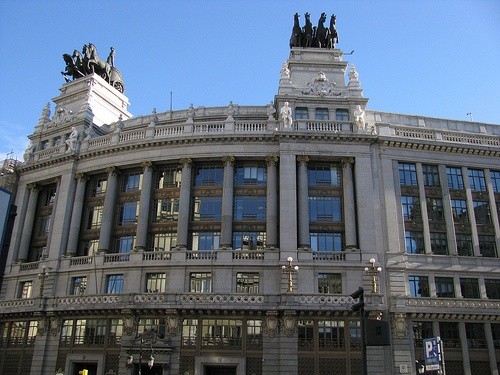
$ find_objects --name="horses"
[289,12,339,50]
[60,43,112,86]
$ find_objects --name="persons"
[107,46,116,68]
[22,140,35,165]
[65,126,79,152]
[280,101,293,129]
[353,105,366,131]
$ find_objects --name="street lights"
[126,338,154,375]
[352,286,368,374]
